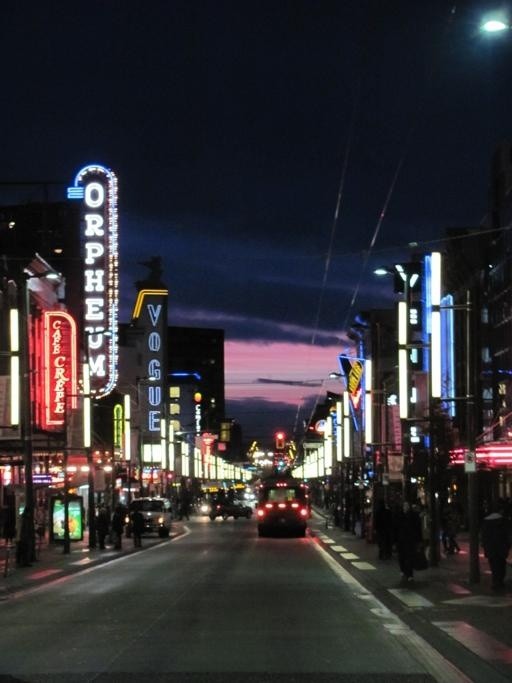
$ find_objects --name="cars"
[125,497,171,538]
[209,497,252,520]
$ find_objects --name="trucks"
[256,482,308,537]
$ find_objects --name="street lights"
[16,269,62,561]
[82,330,113,547]
[371,265,414,502]
[136,375,157,496]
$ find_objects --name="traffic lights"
[275,431,285,449]
[192,392,202,403]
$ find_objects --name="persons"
[179,492,190,520]
[372,499,422,586]
[482,505,509,590]
[442,519,460,555]
[20,512,34,567]
[91,502,146,552]
